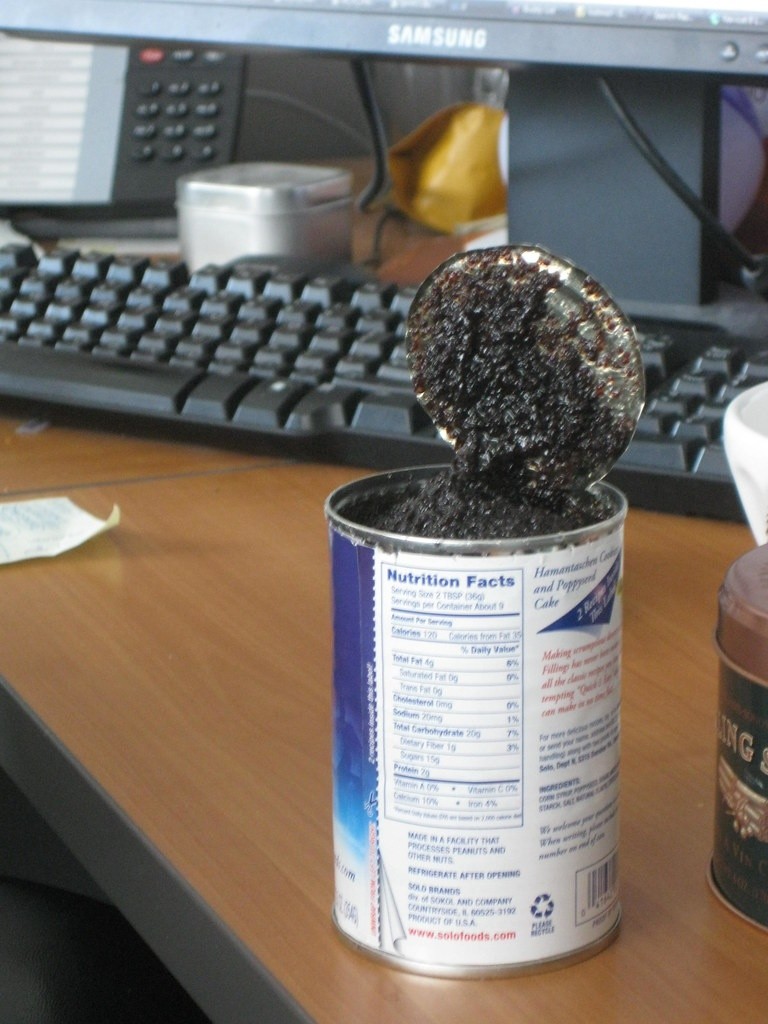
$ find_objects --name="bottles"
[708,515,767,931]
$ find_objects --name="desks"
[0,156,768,1024]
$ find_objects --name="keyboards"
[0,242,768,522]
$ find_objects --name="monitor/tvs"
[0,0,768,313]
